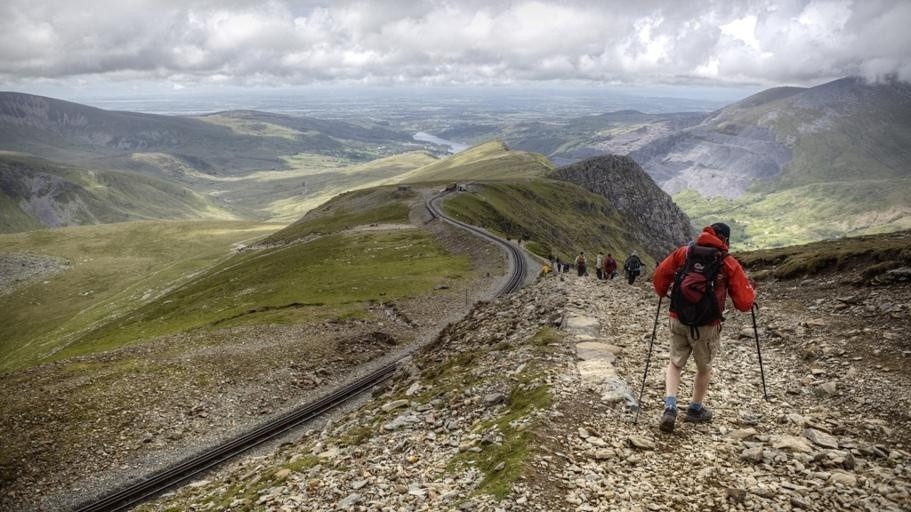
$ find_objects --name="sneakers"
[684,406,714,424]
[660,408,678,432]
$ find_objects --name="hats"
[712,221,731,245]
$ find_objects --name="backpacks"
[670,242,731,326]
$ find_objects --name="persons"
[652,221,756,432]
[549,250,617,280]
[624,249,645,284]
